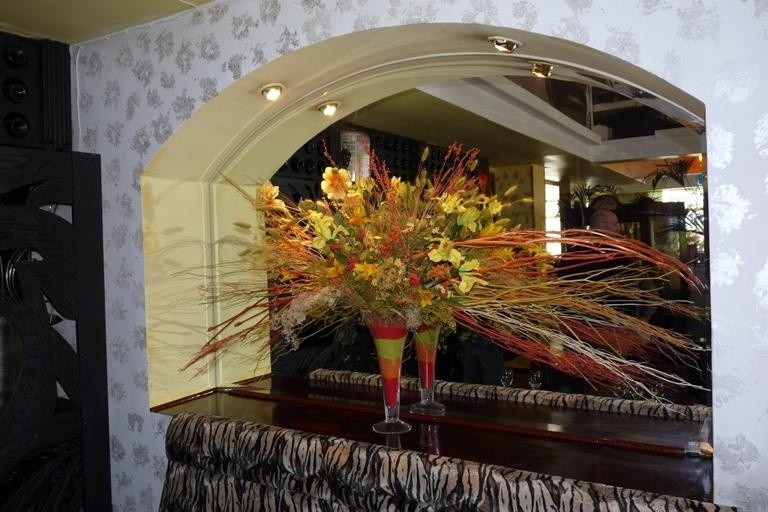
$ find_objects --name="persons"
[630,246,660,325]
[557,210,639,400]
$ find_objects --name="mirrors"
[207,55,710,423]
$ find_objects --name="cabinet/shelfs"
[559,201,689,305]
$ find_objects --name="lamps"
[257,82,286,102]
[315,98,340,120]
[486,36,522,55]
[531,63,554,78]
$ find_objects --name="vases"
[409,322,446,418]
[382,435,402,447]
[360,307,410,435]
[419,423,441,456]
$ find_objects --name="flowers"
[165,137,710,417]
[244,138,712,419]
